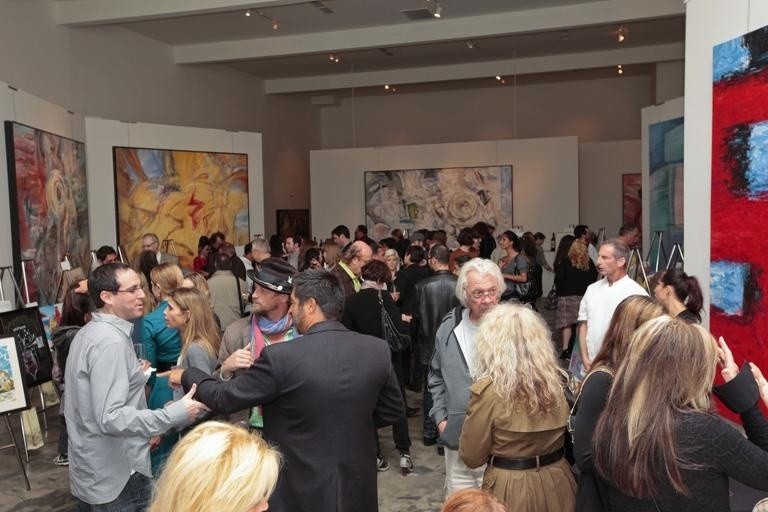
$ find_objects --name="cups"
[134,343,148,360]
[390,285,398,293]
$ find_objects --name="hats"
[247,257,296,295]
[409,233,425,241]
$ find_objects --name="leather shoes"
[560,349,569,359]
[408,406,420,415]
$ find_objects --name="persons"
[52,225,767,512]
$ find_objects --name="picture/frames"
[1,304,54,417]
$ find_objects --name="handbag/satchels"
[514,253,542,299]
[378,288,412,352]
[545,288,559,310]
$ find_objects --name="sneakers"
[53,454,69,466]
[377,457,391,471]
[399,455,413,473]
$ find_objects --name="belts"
[489,446,565,469]
